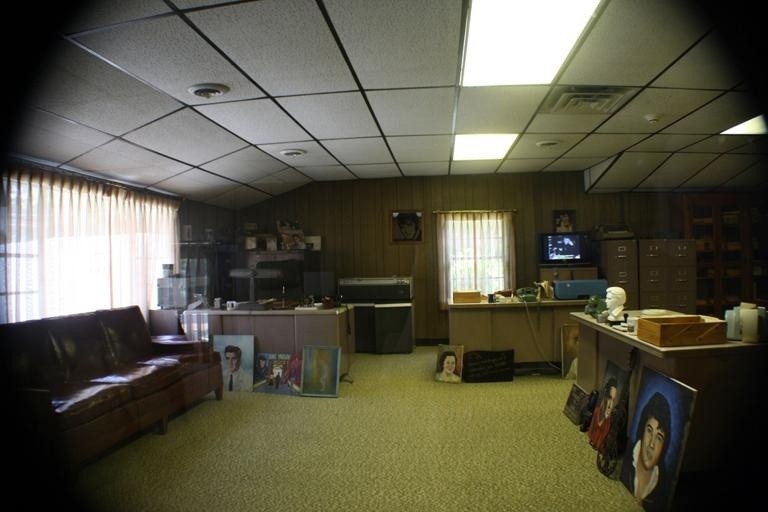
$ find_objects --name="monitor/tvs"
[543,232,586,262]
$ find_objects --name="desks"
[569,307,766,501]
[446,298,607,374]
[183,302,355,379]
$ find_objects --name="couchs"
[0,306,224,474]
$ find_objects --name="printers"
[591,223,634,241]
[553,278,608,300]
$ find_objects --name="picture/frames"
[389,210,425,244]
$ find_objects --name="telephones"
[517,287,538,302]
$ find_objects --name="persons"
[290,234,305,249]
[397,213,422,241]
[605,286,626,321]
[619,393,671,512]
[434,351,460,383]
[319,360,328,391]
[588,379,617,451]
[223,346,251,392]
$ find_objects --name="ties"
[229,375,232,392]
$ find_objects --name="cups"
[227,301,236,311]
[488,294,496,303]
[724,302,759,343]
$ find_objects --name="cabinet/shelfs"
[603,194,767,320]
[338,299,415,354]
